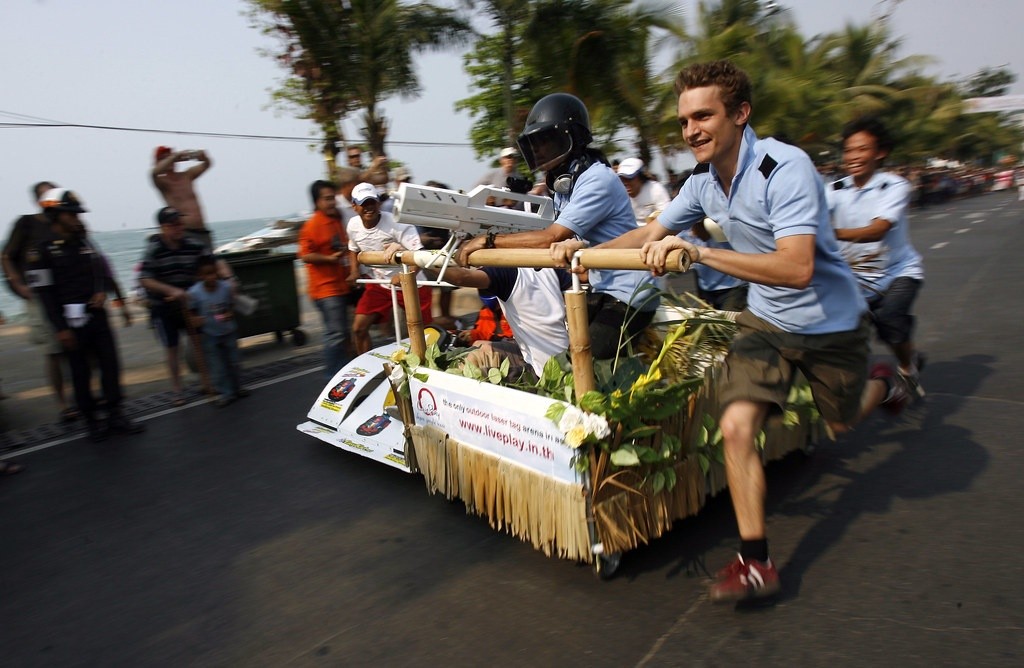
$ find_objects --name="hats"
[158,206,188,223]
[351,182,380,206]
[616,157,645,179]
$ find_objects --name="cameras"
[186,152,199,159]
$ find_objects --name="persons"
[455,94,661,366]
[299,143,749,379]
[813,154,1024,203]
[824,119,927,407]
[136,146,242,406]
[549,62,909,605]
[0,180,144,443]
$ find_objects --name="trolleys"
[295,247,820,582]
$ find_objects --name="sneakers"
[709,555,781,601]
[872,363,909,415]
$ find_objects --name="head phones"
[546,148,599,195]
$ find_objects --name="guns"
[389,175,555,283]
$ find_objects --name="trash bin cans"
[214,248,305,346]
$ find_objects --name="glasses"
[348,154,361,159]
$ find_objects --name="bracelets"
[485,231,499,248]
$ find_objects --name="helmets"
[40,187,88,221]
[516,93,594,174]
